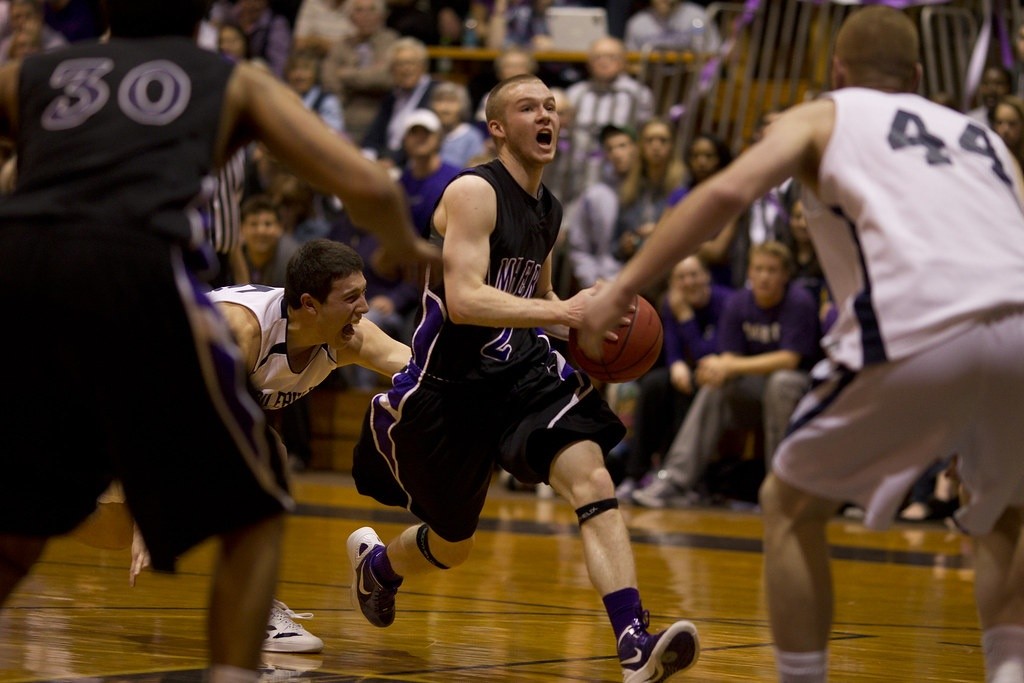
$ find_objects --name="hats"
[399,108,440,140]
[596,125,637,145]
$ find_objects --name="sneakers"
[263,593,323,653]
[347,527,402,628]
[616,610,699,683]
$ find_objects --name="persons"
[1,0,1024,531]
[103,233,413,651]
[344,72,701,683]
[0,0,449,683]
[576,5,1024,683]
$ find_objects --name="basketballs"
[566,293,665,385]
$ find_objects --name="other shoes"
[631,471,699,507]
[898,492,960,525]
